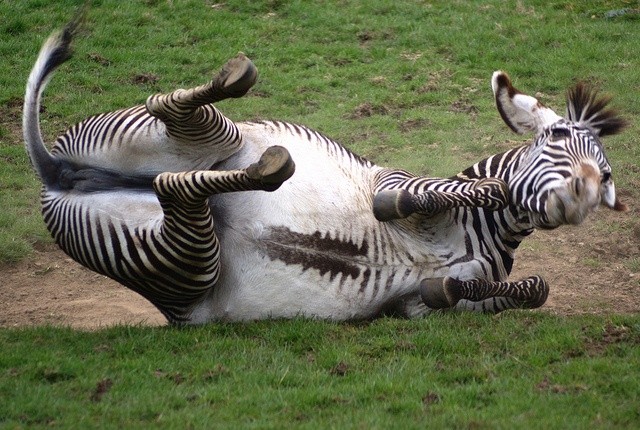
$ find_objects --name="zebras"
[22,14,626,327]
